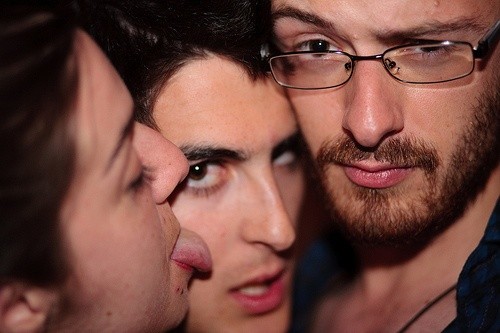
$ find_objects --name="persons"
[0,0,500,333]
[248,0,500,333]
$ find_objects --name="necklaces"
[398,282,457,333]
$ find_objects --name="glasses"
[263,19,500,90]
